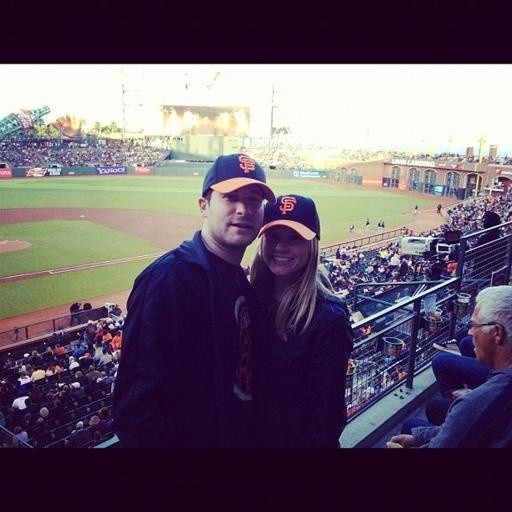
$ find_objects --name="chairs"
[1,334,117,448]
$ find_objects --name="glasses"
[466,321,499,329]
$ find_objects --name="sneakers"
[432,338,462,356]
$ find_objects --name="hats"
[202,153,277,205]
[258,194,320,241]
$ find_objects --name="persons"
[0,134,167,169]
[111,153,277,451]
[248,193,354,451]
[0,302,127,448]
[320,150,512,449]
